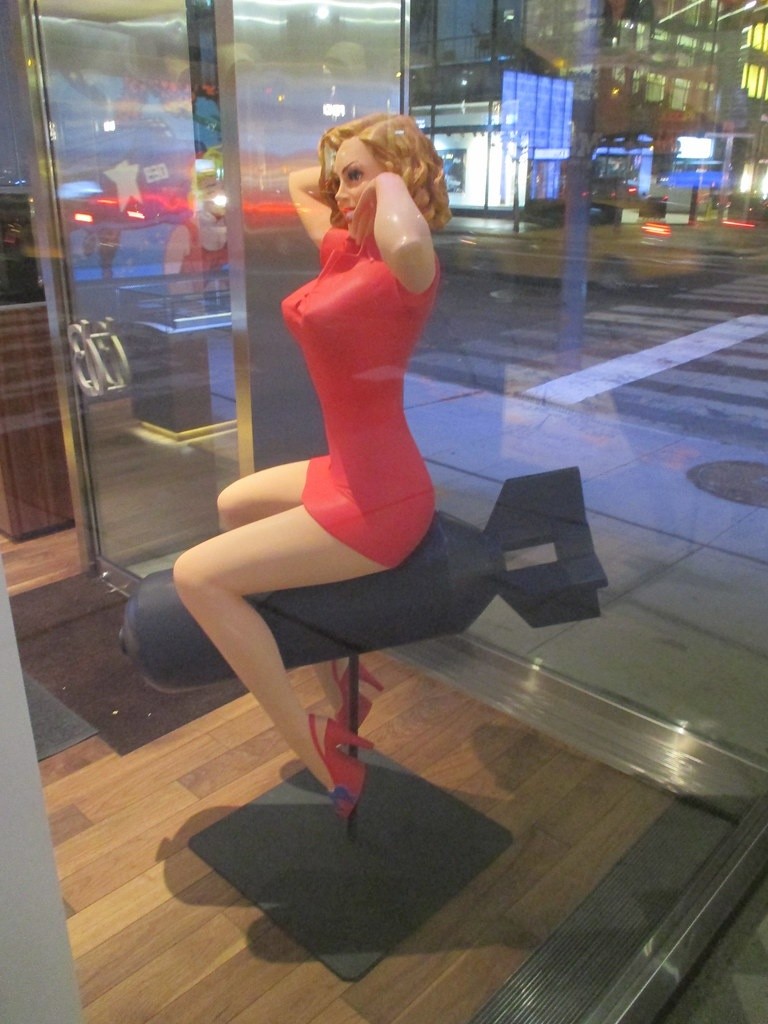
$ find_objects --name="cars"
[454,197,701,295]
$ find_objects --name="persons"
[164,147,229,274]
[173,114,451,819]
